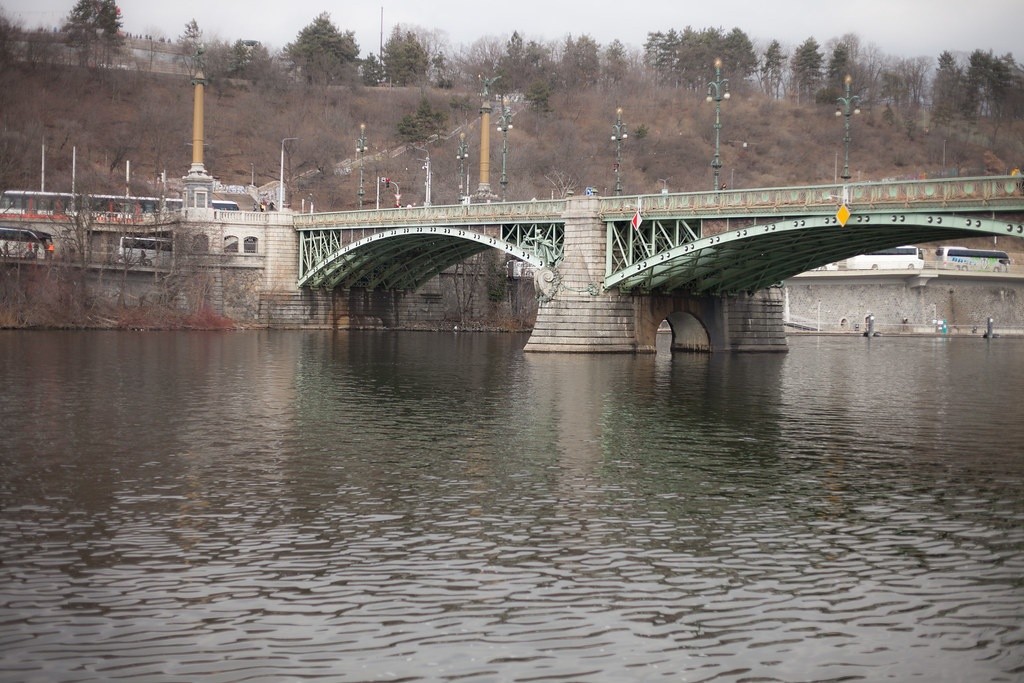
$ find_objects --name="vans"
[120,236,173,266]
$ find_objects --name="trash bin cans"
[936,320,947,332]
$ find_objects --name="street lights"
[611,105,629,197]
[497,115,513,202]
[835,72,861,183]
[706,57,731,194]
[357,123,366,210]
[457,132,469,201]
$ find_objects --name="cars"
[0,227,53,262]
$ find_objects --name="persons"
[28,241,56,259]
[252,201,275,212]
[1012,167,1023,188]
[140,249,146,262]
[718,183,728,197]
[3,241,10,258]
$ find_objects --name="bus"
[936,246,1011,273]
[841,245,925,272]
